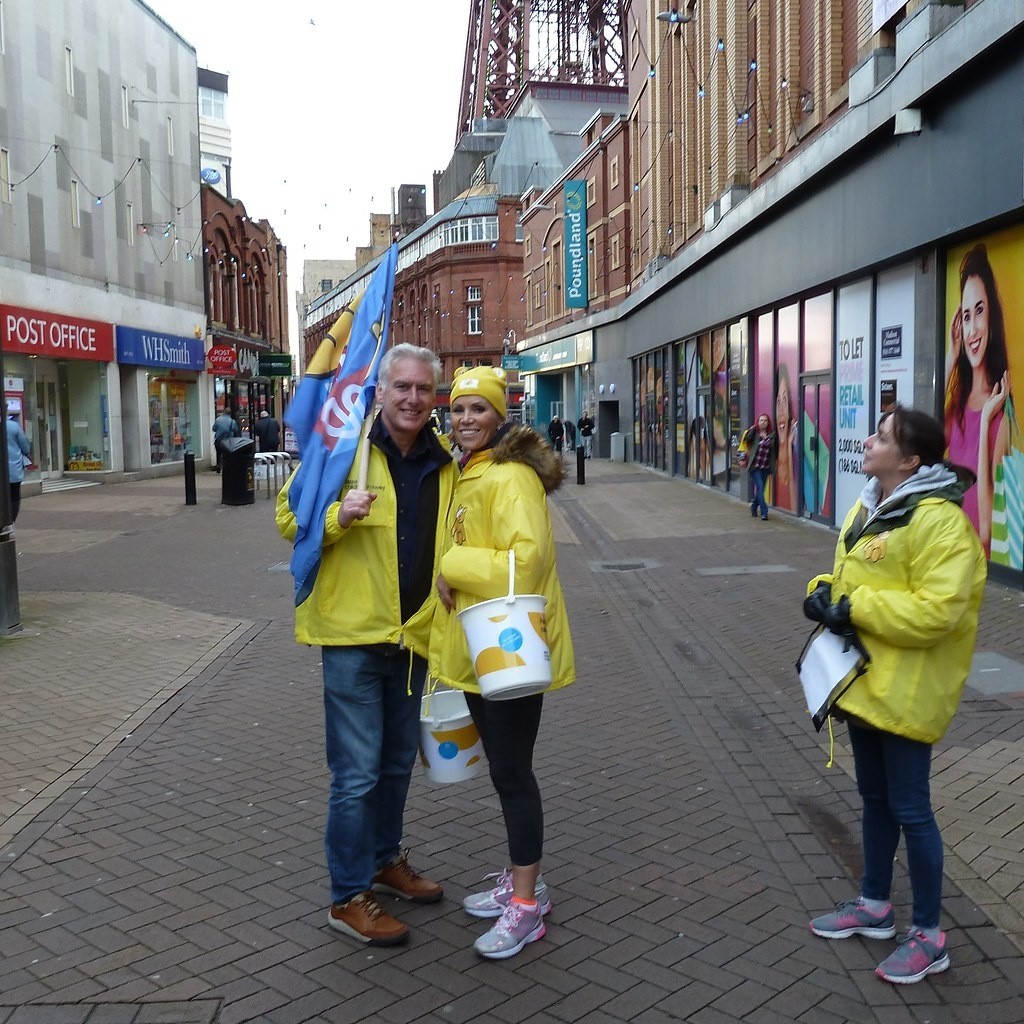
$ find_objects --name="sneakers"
[373,848,443,902]
[462,870,552,916]
[475,900,545,958]
[876,928,950,983]
[809,897,897,940]
[328,892,412,946]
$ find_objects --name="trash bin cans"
[220,437,256,506]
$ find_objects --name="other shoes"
[761,513,768,519]
[751,505,758,517]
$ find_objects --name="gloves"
[825,594,851,632]
[802,582,831,622]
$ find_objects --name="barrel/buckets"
[418,673,483,782]
[456,548,550,701]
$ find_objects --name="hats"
[449,366,508,419]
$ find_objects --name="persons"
[272,343,460,945]
[745,414,772,520]
[5,404,32,530]
[426,365,579,958]
[428,409,463,454]
[768,364,797,514]
[256,411,280,464]
[560,419,576,450]
[213,407,238,472]
[548,415,564,451]
[945,243,1015,561]
[800,404,988,983]
[578,411,595,459]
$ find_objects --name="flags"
[285,242,398,598]
